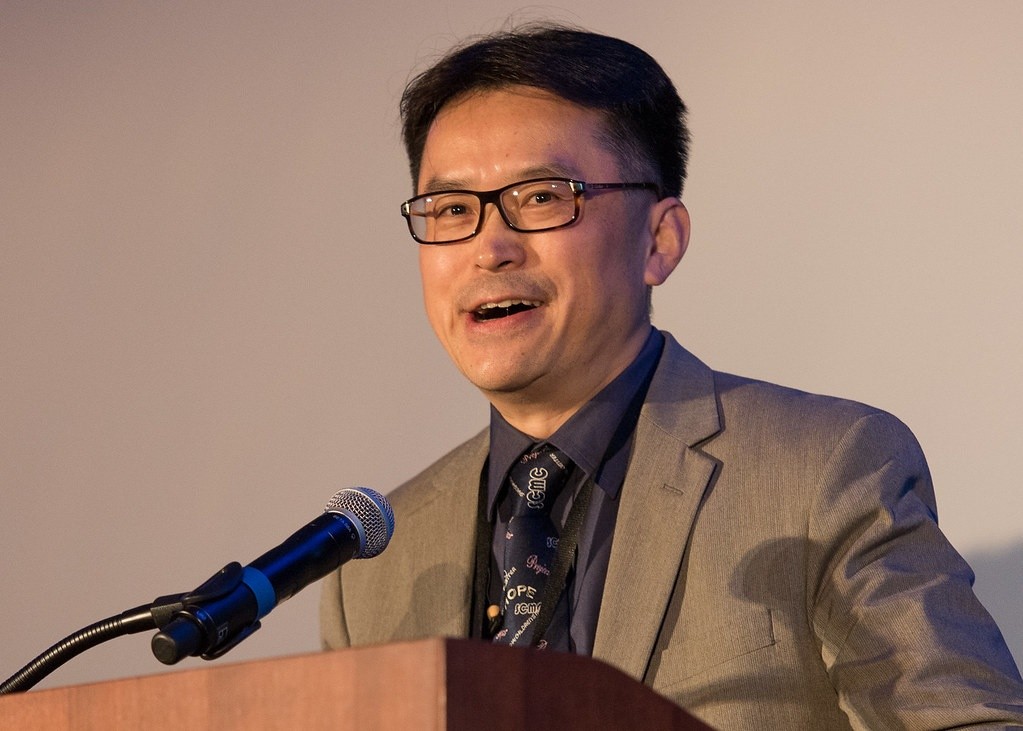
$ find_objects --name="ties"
[488,449,574,653]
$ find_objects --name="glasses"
[398,176,661,245]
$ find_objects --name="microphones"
[153,487,395,669]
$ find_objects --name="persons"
[318,20,1022,731]
